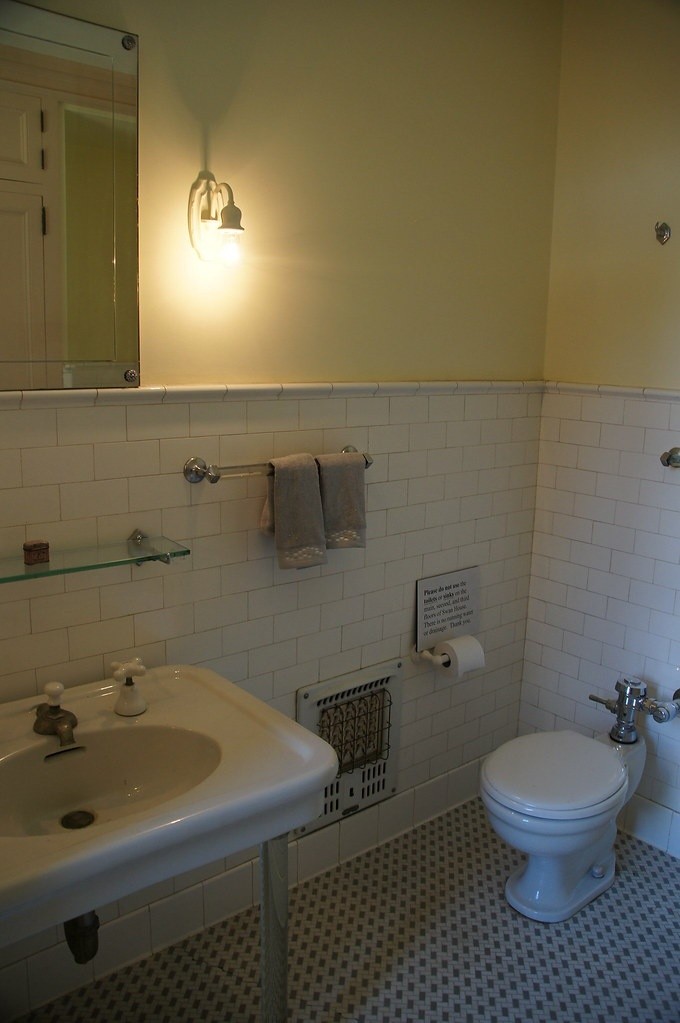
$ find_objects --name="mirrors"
[0,0,143,393]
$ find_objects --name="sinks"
[1,660,341,949]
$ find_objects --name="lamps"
[188,171,245,260]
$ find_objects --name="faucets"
[31,680,78,748]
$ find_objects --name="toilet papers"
[436,633,485,677]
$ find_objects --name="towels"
[312,453,368,550]
[254,453,329,571]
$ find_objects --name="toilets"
[480,728,647,924]
